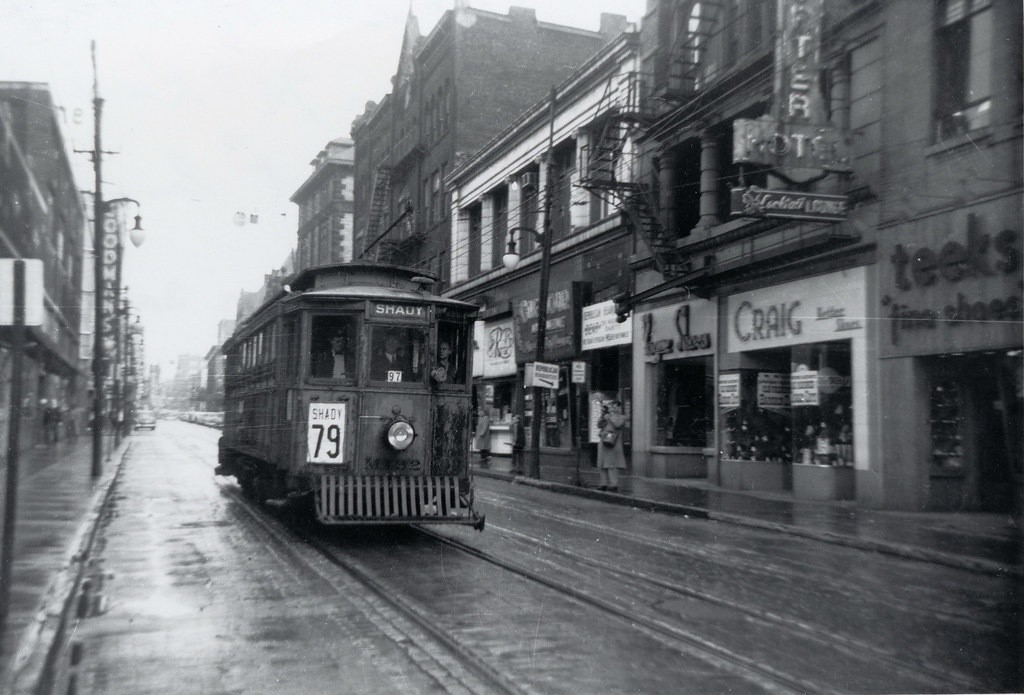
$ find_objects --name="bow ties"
[335,350,343,355]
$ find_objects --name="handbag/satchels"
[602,432,618,447]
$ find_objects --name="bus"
[214,198,487,532]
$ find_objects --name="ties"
[392,354,395,363]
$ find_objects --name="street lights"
[74,98,147,477]
[503,220,553,479]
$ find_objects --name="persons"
[315,329,354,378]
[596,400,627,492]
[43,398,60,444]
[509,415,526,474]
[474,409,492,468]
[108,405,123,433]
[438,342,454,374]
[371,335,412,382]
[64,403,79,445]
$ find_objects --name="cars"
[134,411,156,432]
[182,407,226,427]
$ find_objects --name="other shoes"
[597,485,607,490]
[607,486,617,491]
